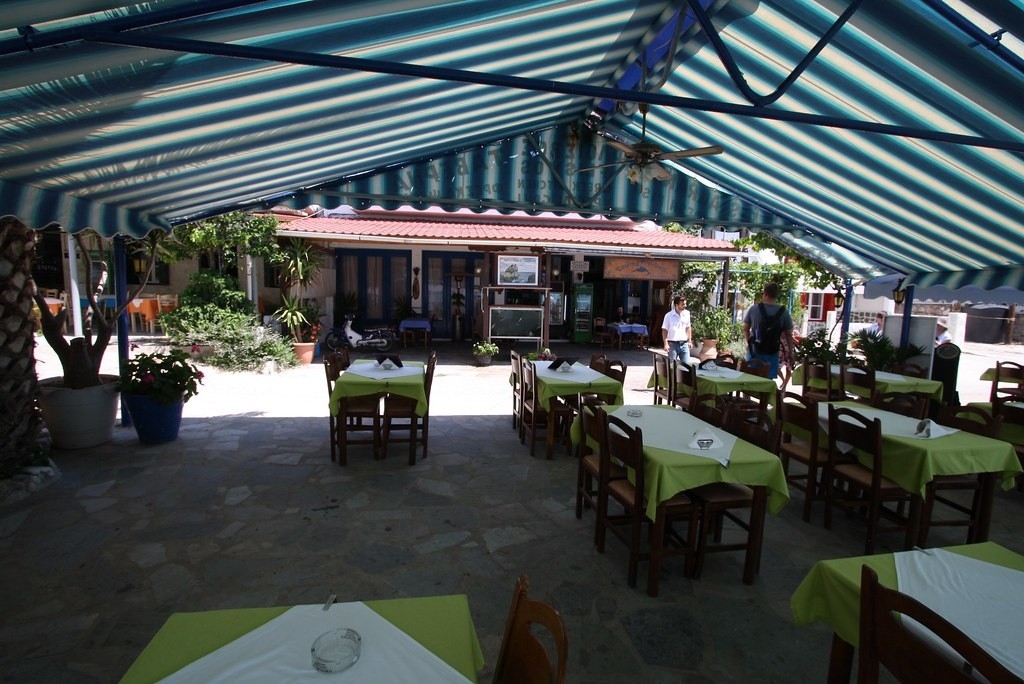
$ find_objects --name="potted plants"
[296,365,297,366]
[32,227,176,451]
[448,291,466,343]
[271,292,320,366]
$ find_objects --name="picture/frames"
[498,255,539,286]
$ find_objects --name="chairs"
[128,291,181,335]
[325,353,1024,684]
[397,314,415,346]
[32,287,73,333]
[592,317,614,350]
[416,314,436,345]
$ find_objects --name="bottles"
[746,353,751,368]
[756,329,759,339]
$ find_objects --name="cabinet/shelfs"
[482,287,553,349]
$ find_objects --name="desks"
[33,297,62,315]
[982,369,1024,384]
[954,402,1024,453]
[117,593,486,684]
[399,315,431,351]
[78,295,115,318]
[128,298,175,334]
[509,359,625,447]
[779,400,1023,549]
[582,403,783,598]
[803,363,943,412]
[647,361,778,448]
[790,539,1024,684]
[605,320,649,351]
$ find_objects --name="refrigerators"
[575,282,593,344]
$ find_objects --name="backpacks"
[748,302,785,355]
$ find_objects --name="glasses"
[878,316,883,318]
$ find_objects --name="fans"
[573,101,724,174]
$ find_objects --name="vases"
[314,342,320,357]
[471,353,500,365]
[120,386,194,446]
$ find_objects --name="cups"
[626,318,630,325]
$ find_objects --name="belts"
[668,339,688,343]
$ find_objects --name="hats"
[937,317,949,331]
[877,311,887,316]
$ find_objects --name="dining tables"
[329,358,430,466]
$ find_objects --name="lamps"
[625,159,674,200]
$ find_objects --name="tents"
[0,0,1024,426]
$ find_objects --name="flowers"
[298,298,328,342]
[117,341,203,403]
[528,347,557,361]
[470,340,500,356]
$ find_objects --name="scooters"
[325,313,399,353]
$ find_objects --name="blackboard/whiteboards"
[489,307,543,339]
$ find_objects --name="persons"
[934,320,951,348]
[744,282,794,400]
[614,305,629,325]
[867,313,884,343]
[662,296,693,365]
